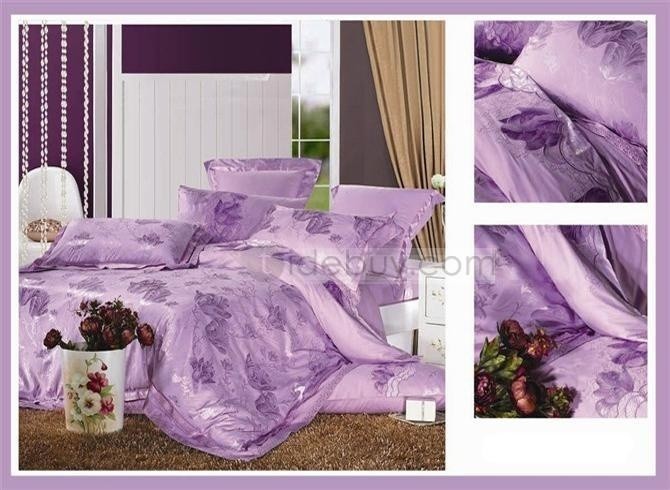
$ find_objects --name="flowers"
[44,298,155,350]
[474,319,575,418]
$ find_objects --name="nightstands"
[418,269,445,370]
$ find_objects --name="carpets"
[19,407,445,470]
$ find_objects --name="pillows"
[320,365,444,413]
[24,220,64,242]
[514,21,647,149]
[239,205,403,292]
[173,184,306,244]
[27,219,210,272]
[204,159,322,209]
[330,185,445,276]
[474,22,537,58]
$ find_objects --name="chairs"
[19,166,84,267]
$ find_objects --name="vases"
[61,350,126,433]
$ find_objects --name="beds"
[20,259,437,461]
